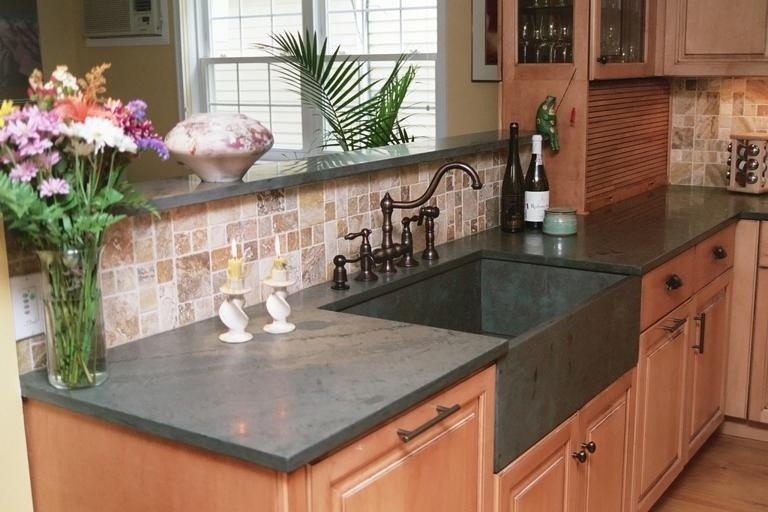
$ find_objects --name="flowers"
[0,61,173,383]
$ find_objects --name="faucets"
[372,162,483,275]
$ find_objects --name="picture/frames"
[469,0,502,84]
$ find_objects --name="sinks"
[318,249,641,472]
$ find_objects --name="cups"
[600,25,637,63]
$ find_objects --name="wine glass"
[518,13,573,62]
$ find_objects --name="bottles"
[523,133,551,234]
[725,139,748,187]
[499,121,525,232]
[542,208,578,237]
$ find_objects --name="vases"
[37,249,110,392]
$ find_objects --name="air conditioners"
[81,0,163,39]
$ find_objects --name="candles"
[272,235,285,269]
[227,240,244,275]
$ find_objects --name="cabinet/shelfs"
[501,1,663,79]
[493,373,639,511]
[732,222,767,440]
[662,0,766,80]
[21,365,494,511]
[639,215,731,511]
